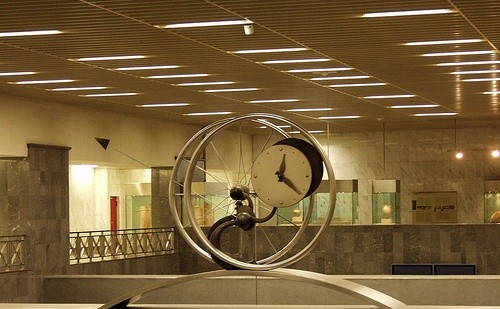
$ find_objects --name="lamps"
[244,24,254,35]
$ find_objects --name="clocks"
[251,144,312,208]
